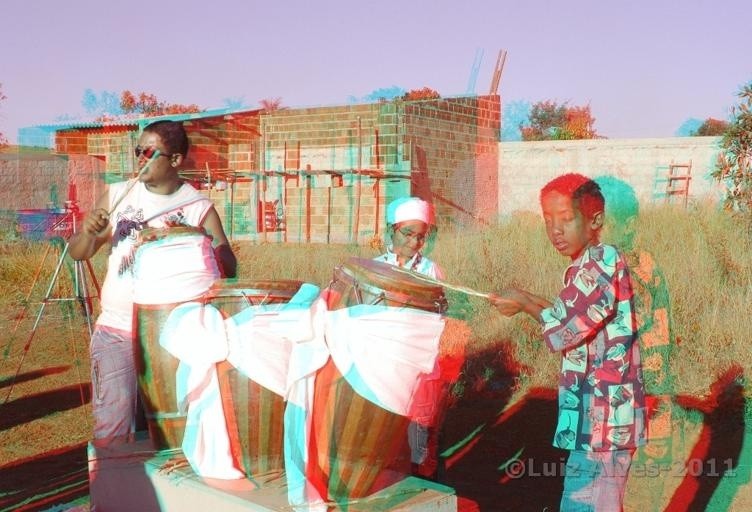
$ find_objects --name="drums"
[205,280,300,478]
[133,272,214,450]
[313,257,447,500]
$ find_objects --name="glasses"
[396,228,425,242]
[134,145,172,160]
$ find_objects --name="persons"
[67,121,238,441]
[373,193,444,279]
[487,172,650,512]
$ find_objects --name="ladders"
[665,159,692,208]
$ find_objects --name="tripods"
[0,239,106,427]
